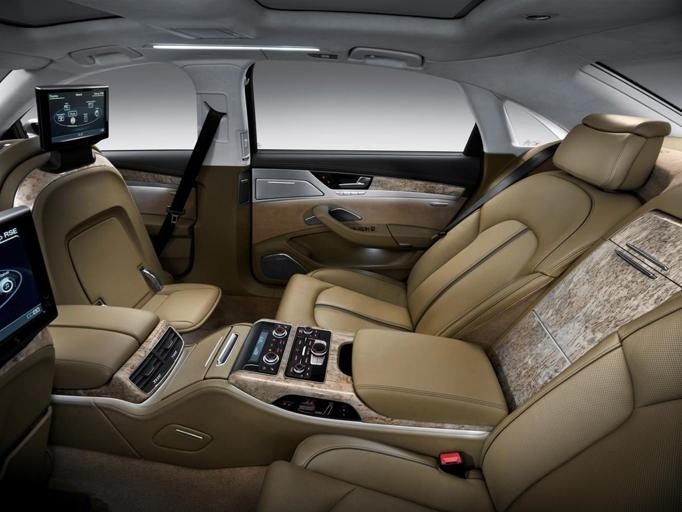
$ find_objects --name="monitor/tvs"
[35,85,109,149]
[0,205,58,369]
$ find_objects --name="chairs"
[0,149,223,333]
[275,112,671,357]
[256,289,682,512]
